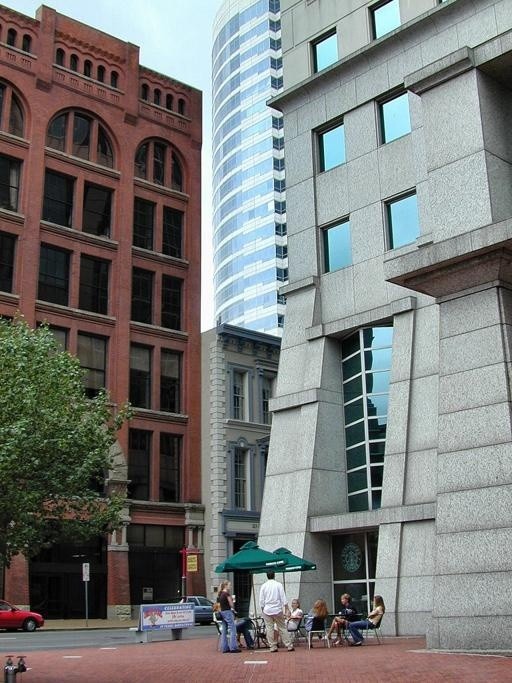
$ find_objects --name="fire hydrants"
[3,656,28,682]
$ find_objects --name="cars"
[0,599,44,632]
[174,595,222,626]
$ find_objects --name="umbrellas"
[215,540,288,582]
[268,547,316,587]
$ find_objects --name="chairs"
[212,609,384,649]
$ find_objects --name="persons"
[304,599,328,648]
[216,580,243,654]
[325,592,357,646]
[259,569,296,652]
[264,597,303,638]
[349,594,386,646]
[214,584,257,650]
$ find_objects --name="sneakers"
[222,648,242,653]
[321,634,363,646]
[271,647,296,652]
